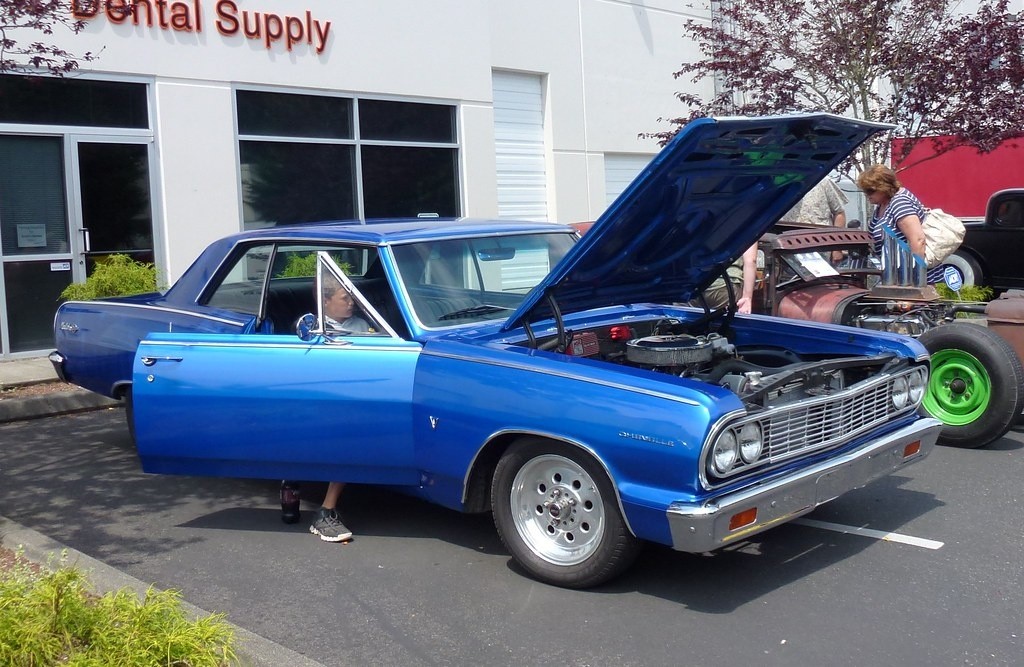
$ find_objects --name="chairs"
[419,297,481,322]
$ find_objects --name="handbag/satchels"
[890,194,967,270]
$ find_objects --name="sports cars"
[46,111,951,590]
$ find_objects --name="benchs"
[242,278,390,334]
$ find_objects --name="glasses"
[862,187,878,195]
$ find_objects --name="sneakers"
[309,505,353,543]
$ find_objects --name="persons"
[294,270,378,541]
[687,238,758,316]
[857,164,944,287]
[777,176,850,267]
